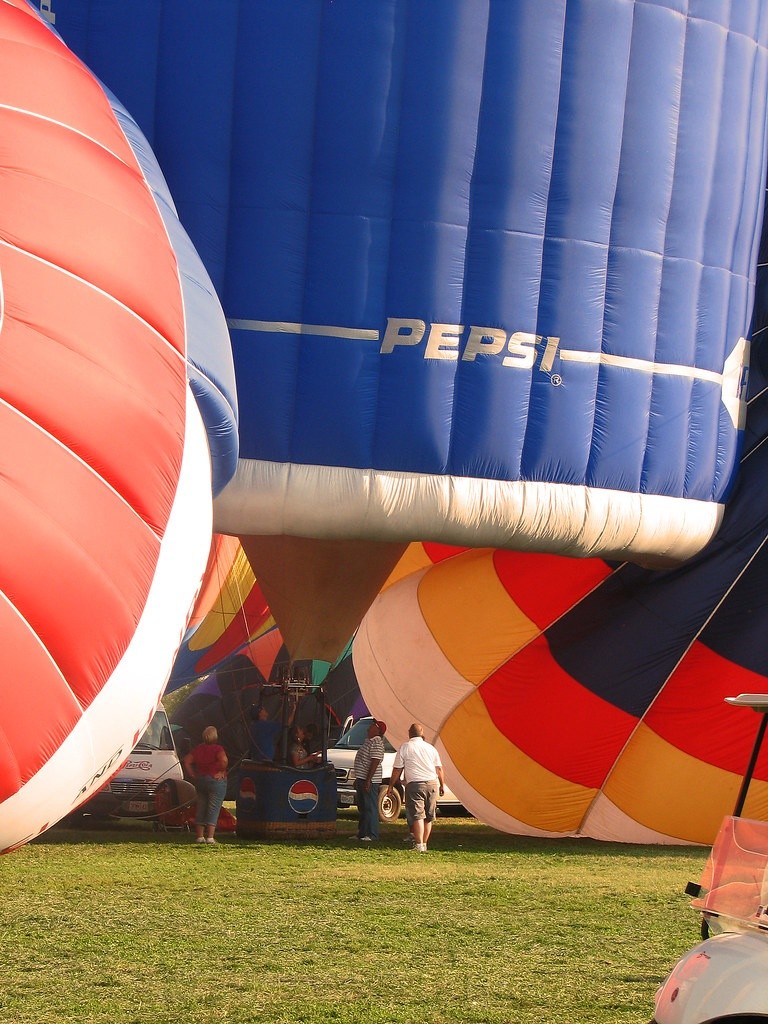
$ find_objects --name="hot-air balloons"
[1,0,241,854]
[30,1,768,845]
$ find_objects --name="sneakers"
[348,835,359,840]
[414,843,427,852]
[206,838,215,844]
[196,837,206,844]
[360,836,372,842]
[404,833,415,843]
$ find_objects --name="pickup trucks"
[310,716,472,823]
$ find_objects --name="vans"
[77,703,186,820]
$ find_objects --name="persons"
[182,725,228,844]
[346,718,387,841]
[286,724,320,770]
[239,701,297,770]
[387,723,444,853]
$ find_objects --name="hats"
[373,718,386,734]
